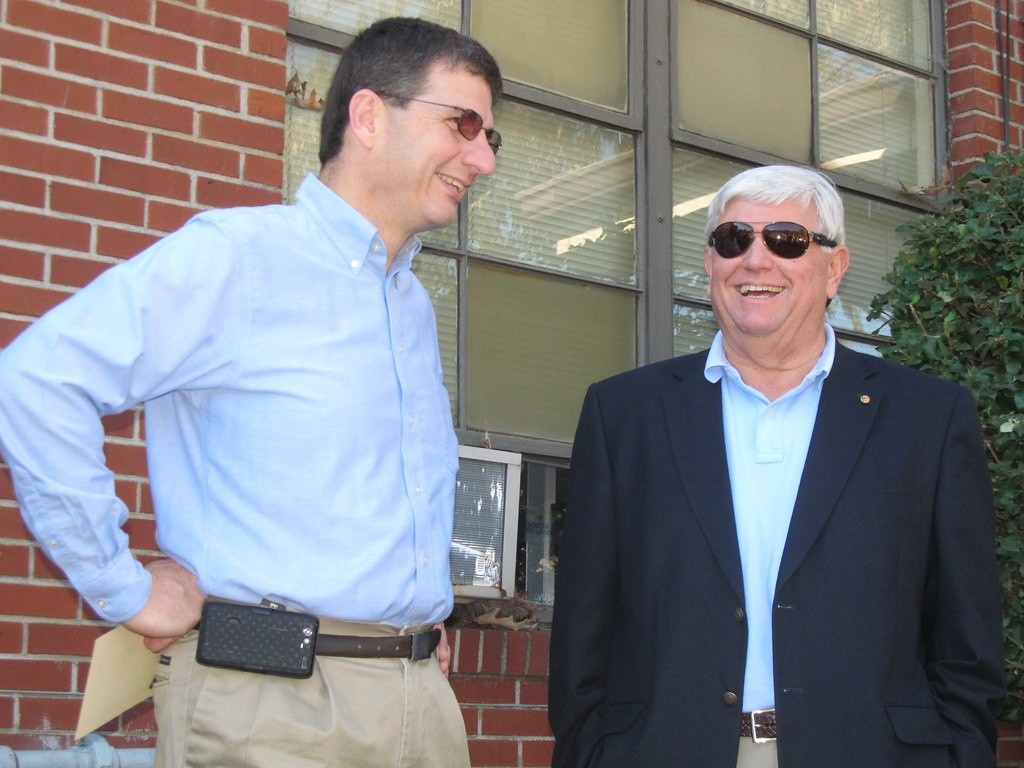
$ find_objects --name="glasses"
[376,91,503,154]
[709,221,836,259]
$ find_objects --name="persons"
[549,165,1003,768]
[0,17,502,768]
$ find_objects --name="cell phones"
[195,600,320,680]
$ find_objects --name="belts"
[193,623,441,661]
[740,708,777,743]
